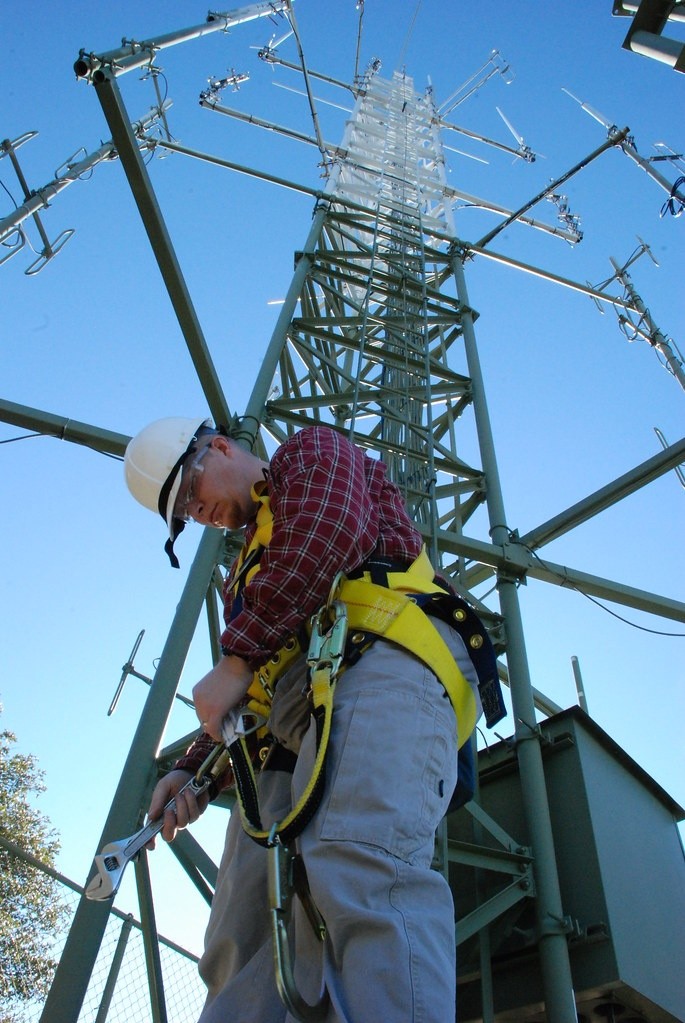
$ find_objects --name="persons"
[125,414,508,1022]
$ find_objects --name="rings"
[200,720,206,728]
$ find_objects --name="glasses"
[176,443,213,524]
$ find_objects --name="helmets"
[123,417,211,542]
